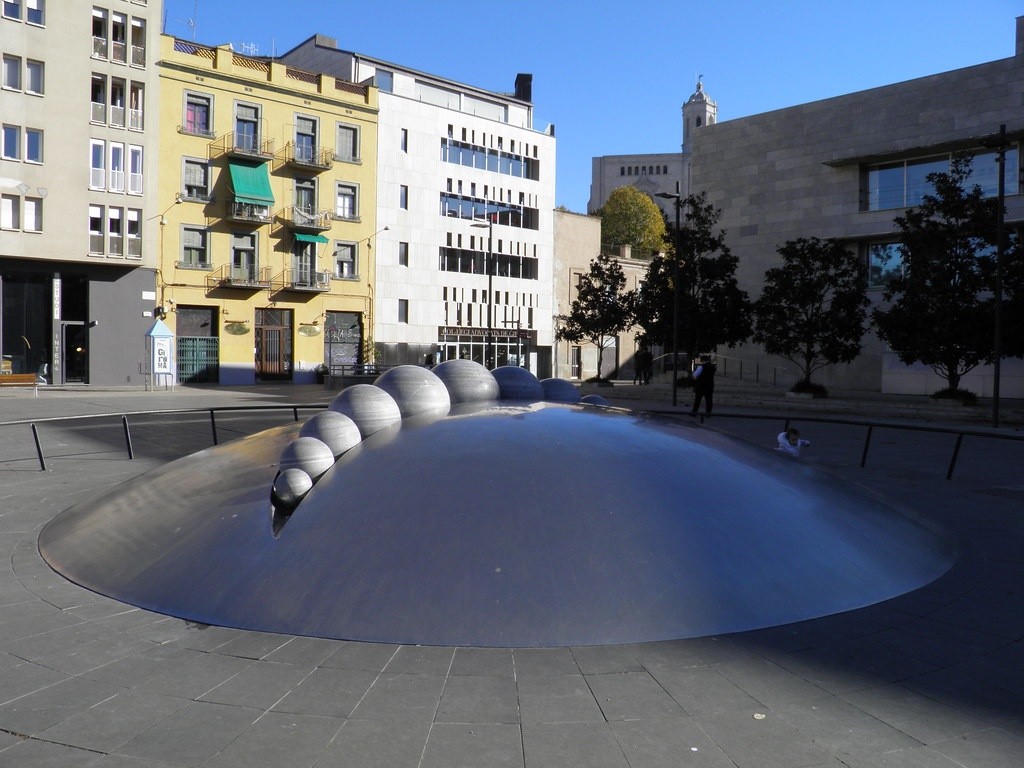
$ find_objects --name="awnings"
[226,156,275,208]
[287,230,329,259]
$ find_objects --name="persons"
[633,346,644,386]
[638,347,654,385]
[774,427,810,457]
[688,356,717,420]
